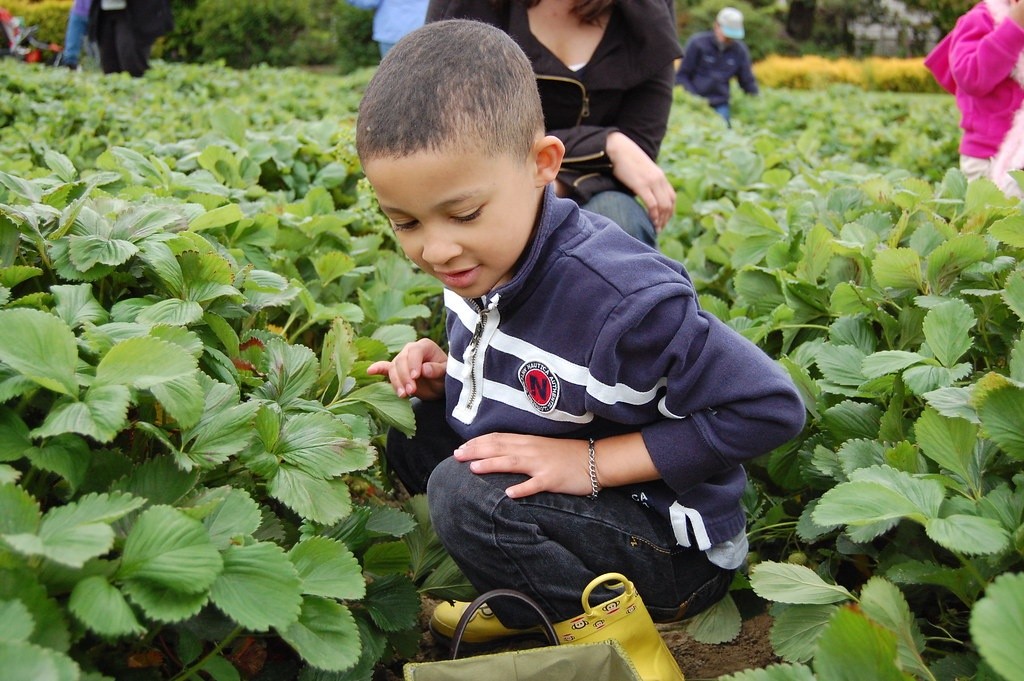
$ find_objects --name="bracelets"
[587,437,600,500]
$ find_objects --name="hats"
[716,7,745,40]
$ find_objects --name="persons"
[925,0,1024,198]
[345,0,428,58]
[64,0,174,77]
[422,0,675,250]
[356,20,808,640]
[675,8,759,125]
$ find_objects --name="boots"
[551,571,684,681]
[428,596,543,644]
